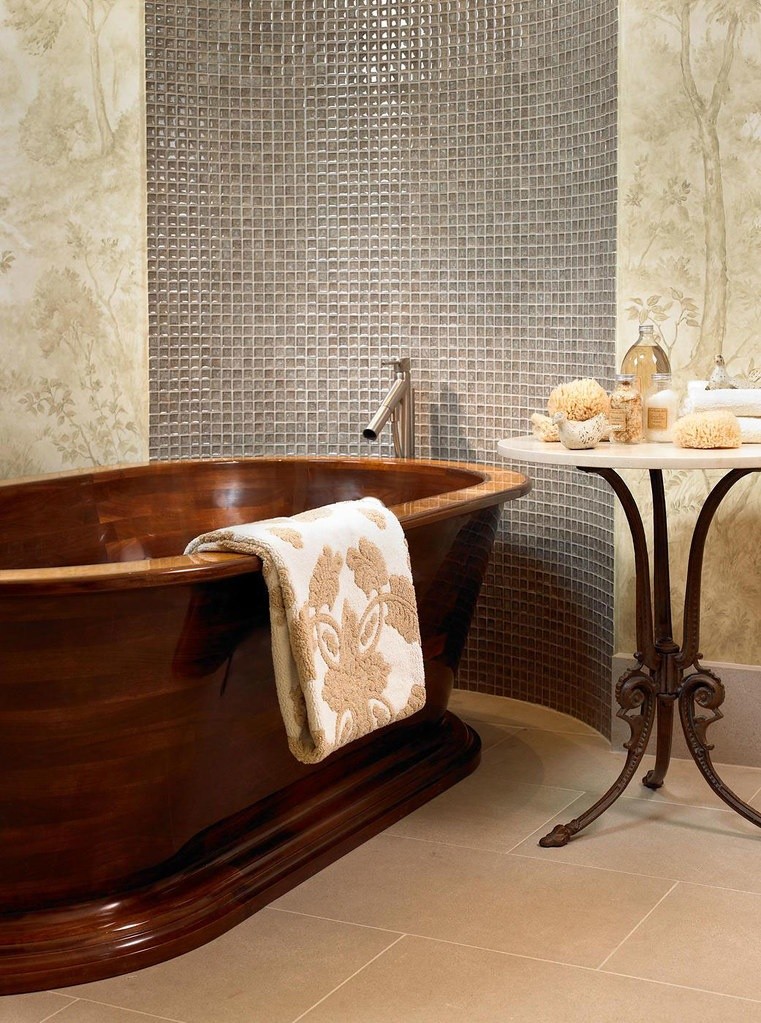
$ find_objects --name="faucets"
[362,357,415,459]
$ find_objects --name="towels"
[183,495,427,765]
[682,388,761,445]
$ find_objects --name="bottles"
[620,325,672,439]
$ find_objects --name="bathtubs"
[0,457,534,998]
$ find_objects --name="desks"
[498,435,761,848]
